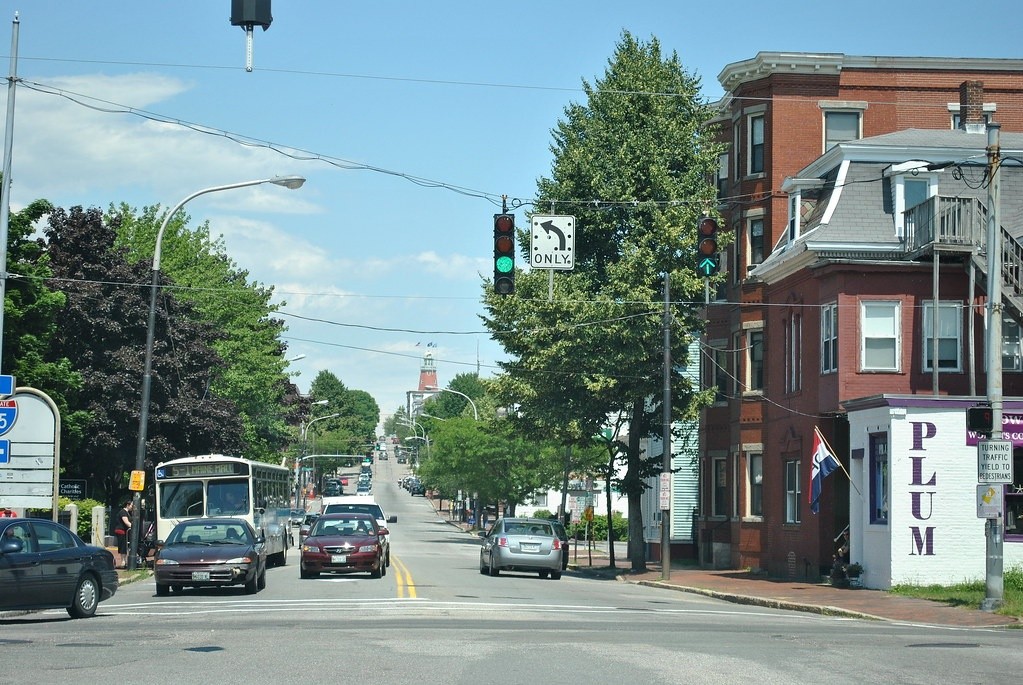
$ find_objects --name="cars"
[0,518,119,618]
[300,513,390,580]
[154,518,268,597]
[291,509,320,549]
[477,518,569,580]
[379,436,426,498]
[321,462,373,497]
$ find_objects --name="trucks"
[321,495,398,566]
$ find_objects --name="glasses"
[843,533,849,536]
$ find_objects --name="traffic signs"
[531,215,575,270]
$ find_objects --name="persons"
[832,530,850,563]
[115,499,133,568]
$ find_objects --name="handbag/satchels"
[115,524,126,535]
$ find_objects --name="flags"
[809,430,840,514]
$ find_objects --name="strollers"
[125,521,155,568]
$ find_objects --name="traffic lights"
[366,452,371,462]
[376,443,380,450]
[494,214,516,295]
[697,218,719,277]
[966,407,993,432]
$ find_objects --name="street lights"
[127,175,307,570]
[425,385,477,421]
[303,413,340,509]
[396,418,425,459]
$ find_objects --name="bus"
[154,454,293,568]
